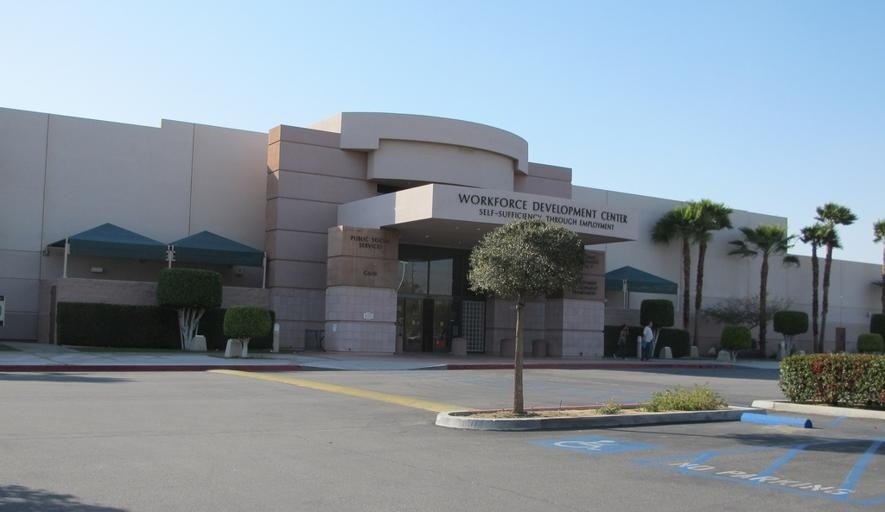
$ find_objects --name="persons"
[641,320,654,360]
[616,323,631,353]
